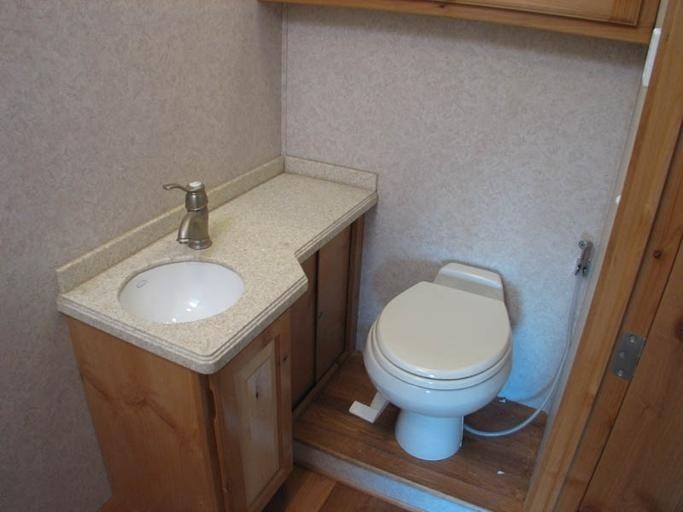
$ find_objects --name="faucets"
[161,180,213,252]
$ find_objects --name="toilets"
[360,260,516,463]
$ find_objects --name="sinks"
[115,256,248,326]
